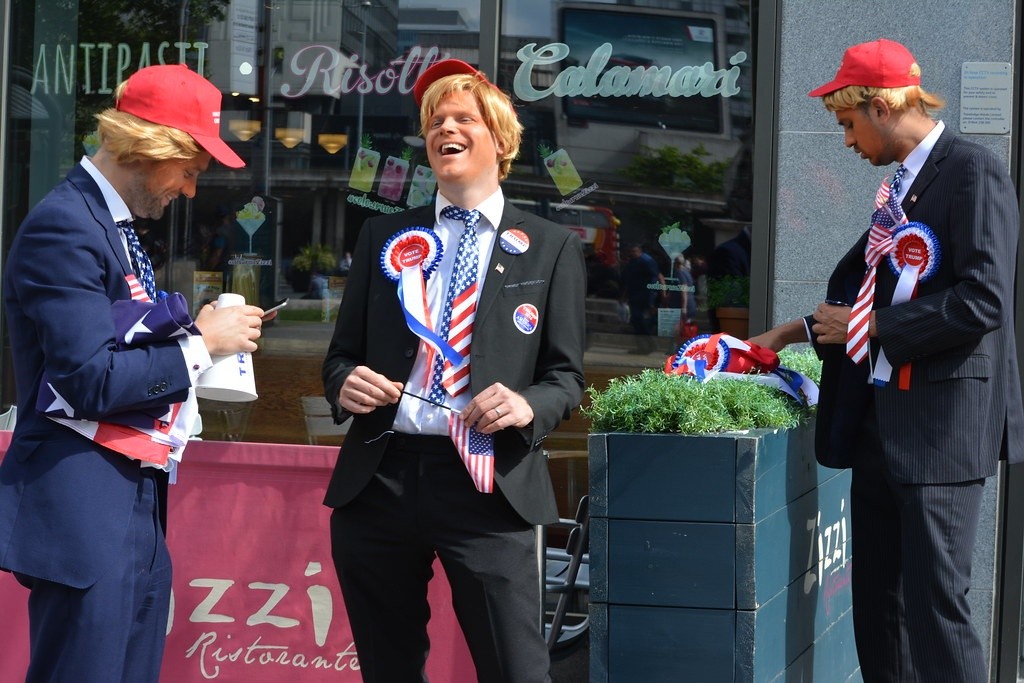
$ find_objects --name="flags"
[448,411,495,493]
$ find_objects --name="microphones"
[263,298,289,317]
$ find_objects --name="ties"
[115,220,157,307]
[426,205,483,409]
[846,164,909,365]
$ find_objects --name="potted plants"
[579,345,864,683]
[707,275,749,343]
[291,244,339,297]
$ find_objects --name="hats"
[808,40,923,98]
[413,58,502,108]
[116,65,246,169]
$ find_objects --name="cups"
[190,293,259,404]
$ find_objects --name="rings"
[495,408,501,418]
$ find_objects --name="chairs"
[542,496,591,660]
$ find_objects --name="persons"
[284,250,329,301]
[322,59,586,683]
[136,222,161,261]
[337,252,352,277]
[0,63,277,683]
[199,216,227,293]
[707,172,754,334]
[620,243,709,358]
[746,38,1024,683]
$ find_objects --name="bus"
[550,0,747,216]
